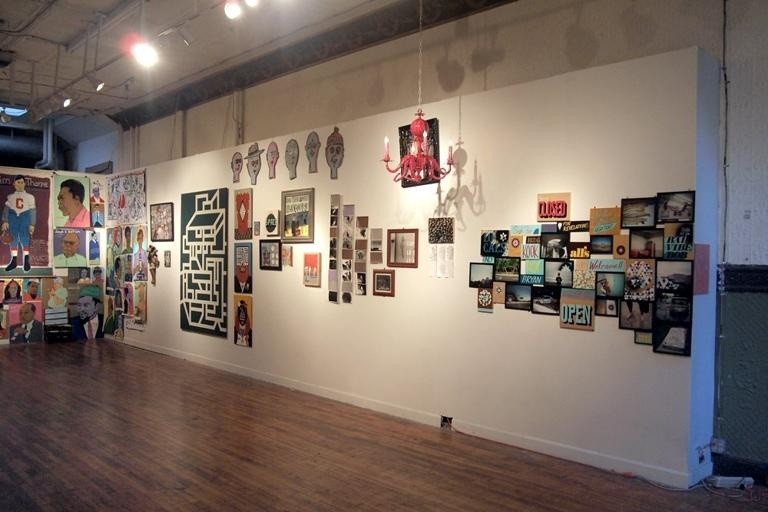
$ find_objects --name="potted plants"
[373,269,397,296]
[148,202,176,241]
[384,229,420,268]
[259,239,284,269]
[468,190,694,357]
[280,188,316,245]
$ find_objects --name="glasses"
[383,1,454,184]
[51,45,71,107]
[82,22,107,91]
